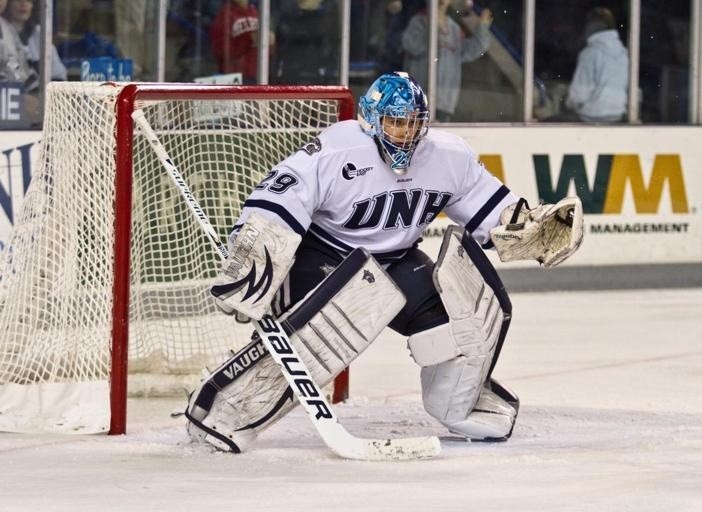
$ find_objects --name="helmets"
[357,72,430,170]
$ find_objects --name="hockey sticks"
[130,109,442,461]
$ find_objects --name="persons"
[0,1,68,93]
[564,8,631,122]
[402,2,492,122]
[185,72,583,452]
[168,0,415,87]
